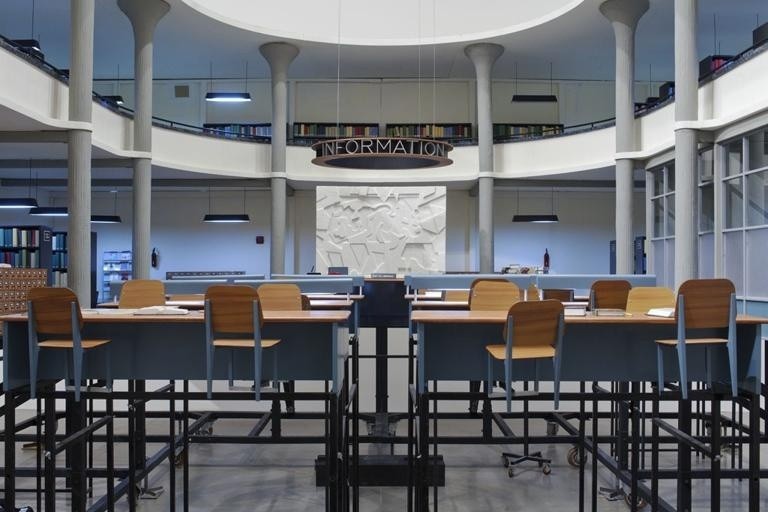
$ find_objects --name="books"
[386,125,471,138]
[203,125,271,137]
[133,305,189,316]
[645,306,675,318]
[493,125,563,145]
[103,251,133,301]
[595,307,624,317]
[563,306,587,317]
[0,227,39,268]
[293,124,379,147]
[51,233,68,288]
[711,59,727,74]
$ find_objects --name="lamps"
[205,62,253,101]
[29,168,70,216]
[0,158,39,208]
[511,60,558,103]
[11,0,41,50]
[103,64,125,104]
[201,182,251,224]
[512,185,561,223]
[91,191,122,223]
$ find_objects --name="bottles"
[544,248,549,273]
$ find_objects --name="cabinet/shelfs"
[0,224,98,307]
